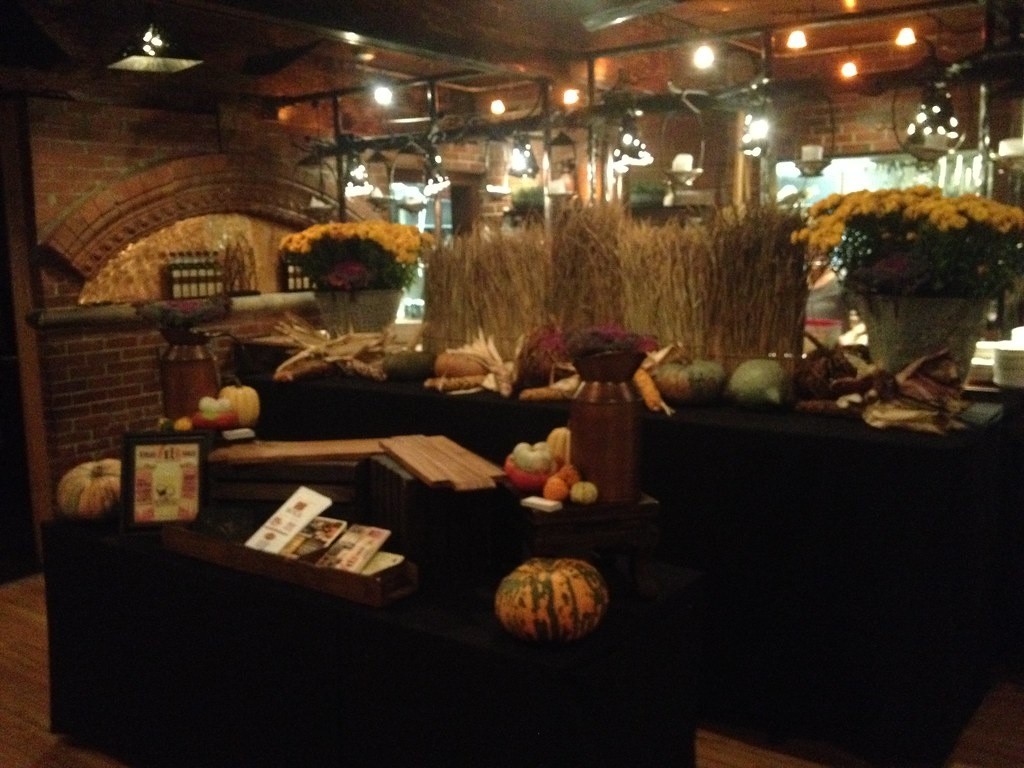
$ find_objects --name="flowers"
[279,219,435,290]
[518,322,575,370]
[789,184,1024,299]
[563,322,661,360]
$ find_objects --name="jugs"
[157,323,250,421]
[566,350,649,504]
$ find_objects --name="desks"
[242,368,1011,768]
[41,516,708,768]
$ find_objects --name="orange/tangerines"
[544,478,568,500]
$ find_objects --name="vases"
[863,294,992,388]
[573,354,647,383]
[312,288,404,335]
[519,368,576,390]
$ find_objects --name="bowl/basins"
[967,326,1024,390]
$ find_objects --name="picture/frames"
[119,428,213,539]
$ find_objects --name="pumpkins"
[435,353,487,375]
[56,458,120,520]
[729,359,803,408]
[217,376,259,427]
[657,358,726,404]
[548,427,570,463]
[495,556,609,643]
[383,351,434,377]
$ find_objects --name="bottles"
[283,259,318,292]
[167,248,222,300]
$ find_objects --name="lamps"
[422,149,451,197]
[507,132,539,181]
[105,18,204,75]
[608,115,654,172]
[345,155,373,200]
[904,72,968,163]
